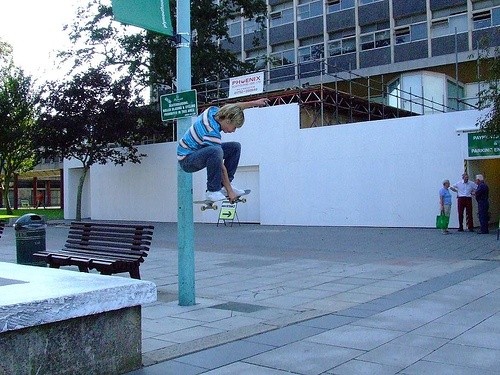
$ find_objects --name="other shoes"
[220,184,245,196]
[458,229,464,232]
[205,190,226,201]
[441,232,447,235]
[478,230,489,234]
[469,229,474,232]
[447,231,454,234]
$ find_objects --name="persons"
[176,97,272,201]
[470,174,489,234]
[450,173,479,232]
[439,180,454,235]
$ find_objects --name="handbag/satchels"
[436,210,449,229]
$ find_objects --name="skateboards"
[194,190,251,211]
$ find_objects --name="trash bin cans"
[14,213,47,267]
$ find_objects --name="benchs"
[32,218,155,280]
